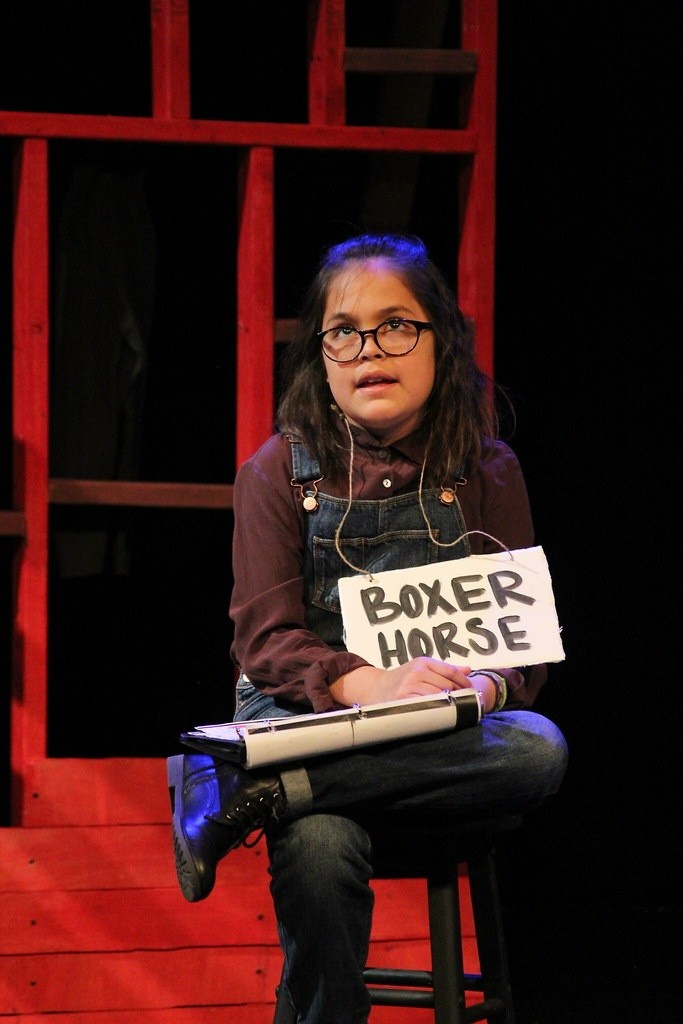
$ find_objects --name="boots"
[167,754,286,902]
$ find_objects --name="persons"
[167,236,567,1024]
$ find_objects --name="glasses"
[317,319,432,363]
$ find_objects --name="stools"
[274,808,522,1024]
